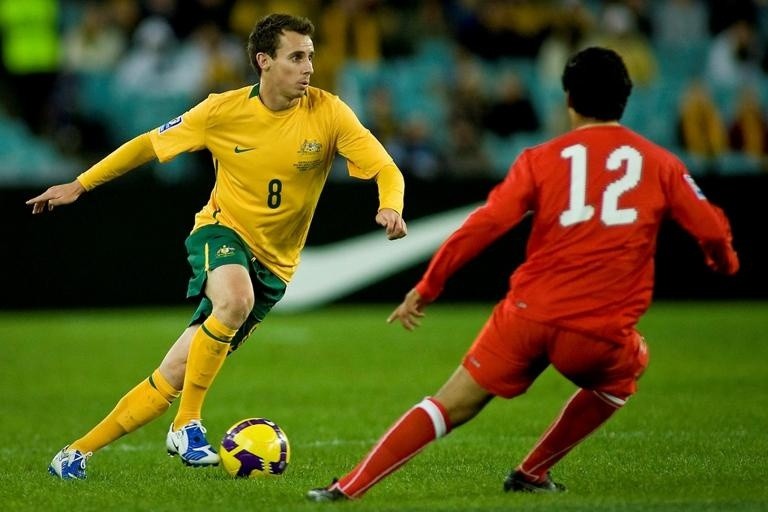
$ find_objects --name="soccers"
[219,417,292,477]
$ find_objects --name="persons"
[23,9,406,484]
[304,46,739,504]
[0,0,767,177]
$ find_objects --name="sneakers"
[164,421,221,468]
[502,470,568,492]
[47,444,91,481]
[305,476,352,506]
